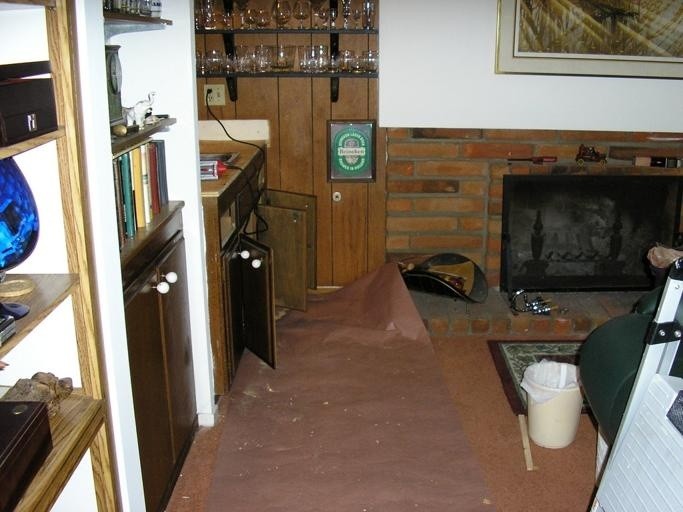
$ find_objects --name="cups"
[195,44,378,75]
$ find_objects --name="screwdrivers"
[508,156,557,162]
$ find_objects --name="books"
[111,138,169,251]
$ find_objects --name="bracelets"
[0,279,35,298]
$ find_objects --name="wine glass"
[194,0,375,33]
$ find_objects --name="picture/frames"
[493,0,683,81]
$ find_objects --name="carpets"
[486,339,592,418]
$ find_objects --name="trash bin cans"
[520,360,584,449]
